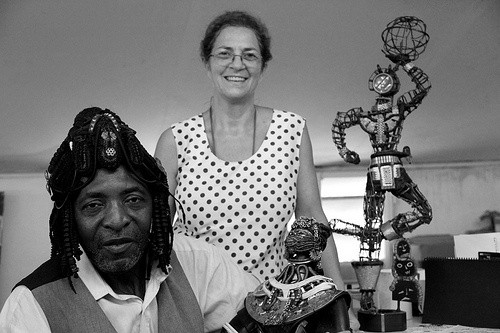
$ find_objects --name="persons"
[329,48,434,303]
[152,7,362,333]
[217,215,356,332]
[0,103,261,333]
[389,234,425,317]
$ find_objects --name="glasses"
[207,51,262,62]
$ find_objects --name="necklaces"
[208,103,257,162]
